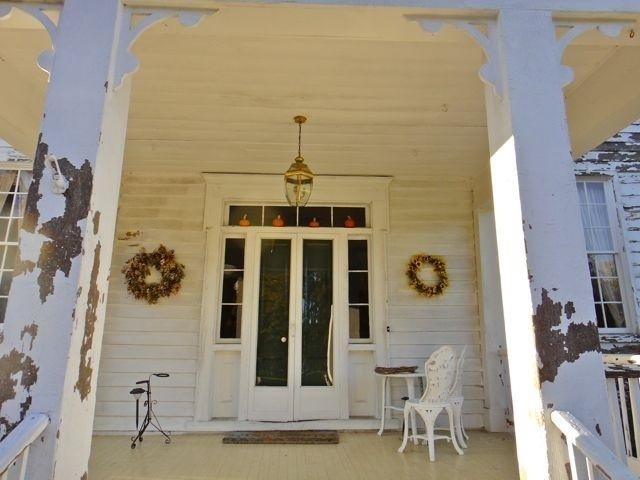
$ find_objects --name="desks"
[377,371,430,443]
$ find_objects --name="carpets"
[222,430,340,444]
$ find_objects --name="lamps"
[239,214,251,226]
[308,218,320,227]
[344,216,356,227]
[285,115,315,206]
[272,215,285,227]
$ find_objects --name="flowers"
[123,244,185,305]
[407,252,449,296]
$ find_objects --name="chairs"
[398,344,465,461]
[434,345,470,448]
